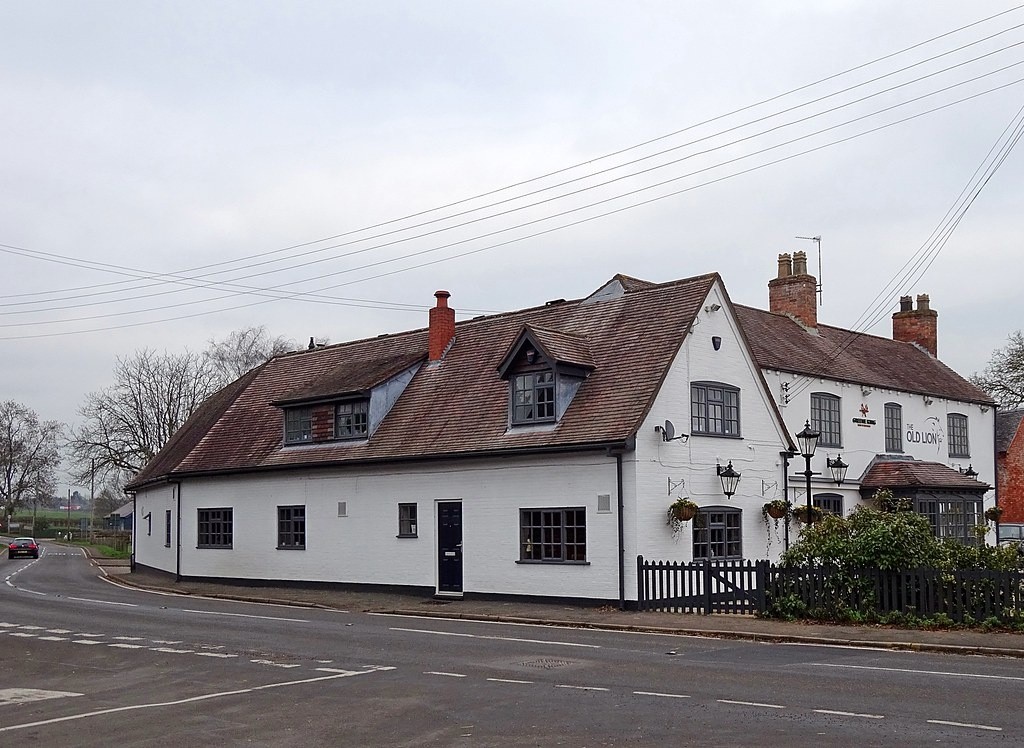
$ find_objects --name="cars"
[8,537,40,559]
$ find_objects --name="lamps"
[861,386,871,397]
[705,303,721,313]
[717,461,741,499]
[923,396,933,406]
[795,419,821,458]
[959,464,979,480]
[827,454,849,487]
[980,405,989,412]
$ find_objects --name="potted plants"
[666,496,704,544]
[762,500,793,557]
[985,506,1003,533]
[793,504,823,536]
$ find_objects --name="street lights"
[796,418,823,530]
